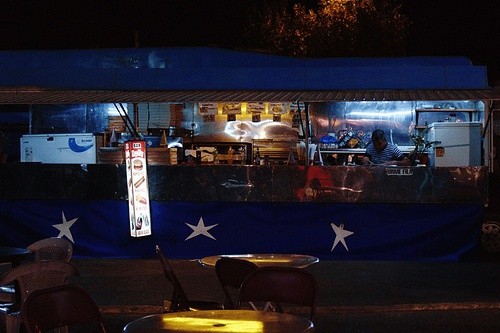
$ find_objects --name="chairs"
[22,286,109,333]
[27,238,72,263]
[0,260,73,333]
[156,245,223,311]
[215,258,317,321]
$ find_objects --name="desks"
[122,311,313,333]
[199,254,319,310]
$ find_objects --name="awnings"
[0,89,500,144]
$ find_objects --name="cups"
[196,146,201,164]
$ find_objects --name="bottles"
[136,212,143,230]
[256,146,260,165]
[240,146,246,165]
[213,145,219,164]
[110,128,116,147]
[227,144,234,165]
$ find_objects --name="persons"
[363,129,411,166]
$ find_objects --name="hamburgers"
[133,160,143,170]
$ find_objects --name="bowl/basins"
[144,137,161,148]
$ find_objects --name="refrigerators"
[421,122,481,167]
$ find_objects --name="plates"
[271,106,284,114]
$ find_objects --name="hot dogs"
[134,176,145,188]
